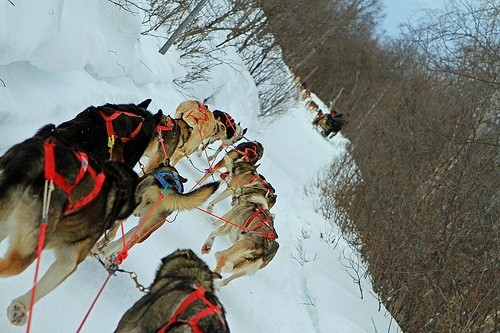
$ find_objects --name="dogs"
[171,100,226,167]
[139,112,194,177]
[202,187,280,290]
[207,159,277,213]
[90,158,221,268]
[292,75,324,117]
[196,139,264,185]
[111,248,230,333]
[197,110,248,161]
[0,98,163,327]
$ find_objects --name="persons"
[329,112,350,140]
